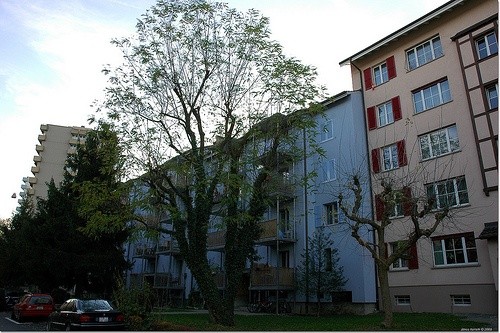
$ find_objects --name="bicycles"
[246,301,291,314]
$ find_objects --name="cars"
[11,293,54,322]
[46,298,125,331]
